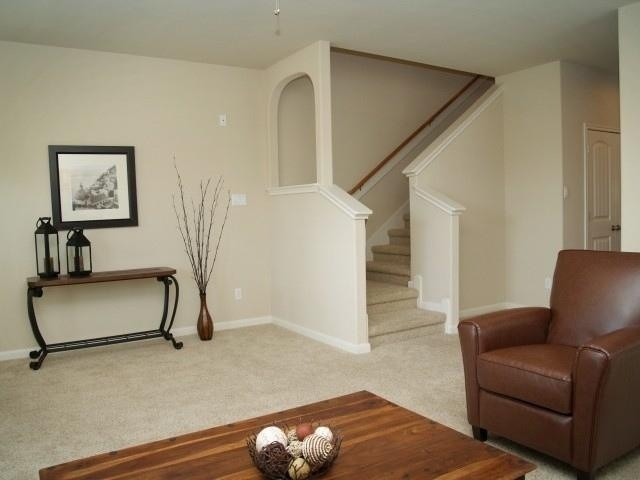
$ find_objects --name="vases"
[197,294,214,341]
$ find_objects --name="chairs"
[457,248,640,479]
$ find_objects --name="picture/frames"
[47,144,139,230]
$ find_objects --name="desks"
[25,266,184,370]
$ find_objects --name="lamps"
[32,215,94,279]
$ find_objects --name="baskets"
[245,416,343,480]
